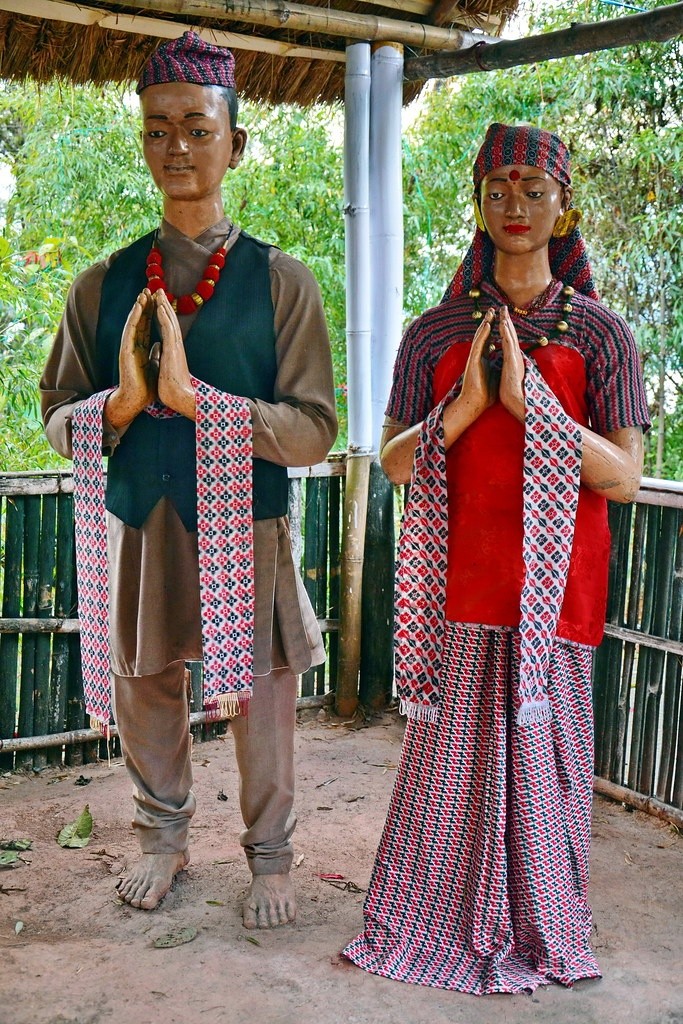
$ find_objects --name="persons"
[339,124,645,996]
[41,32,339,928]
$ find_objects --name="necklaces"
[146,227,233,315]
[468,284,574,352]
